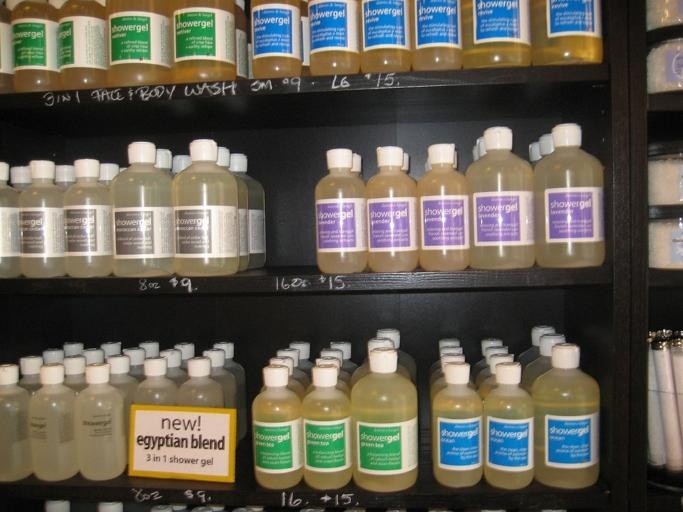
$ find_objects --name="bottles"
[312,123,683,273]
[0,342,244,482]
[0,0,683,94]
[0,138,265,277]
[251,326,682,491]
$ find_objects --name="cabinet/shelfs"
[643,92,683,493]
[0,61,614,512]
